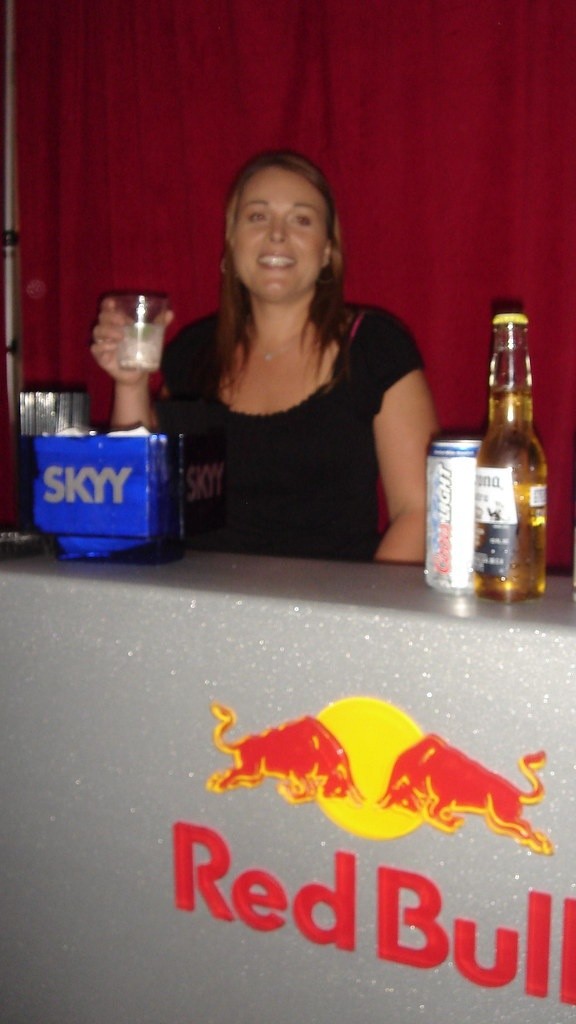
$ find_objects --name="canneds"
[421,439,483,593]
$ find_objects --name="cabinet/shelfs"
[0,547,576,1024]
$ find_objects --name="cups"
[116,295,166,371]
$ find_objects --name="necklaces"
[258,350,277,360]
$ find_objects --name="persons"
[90,148,442,564]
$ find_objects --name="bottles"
[474,313,548,603]
